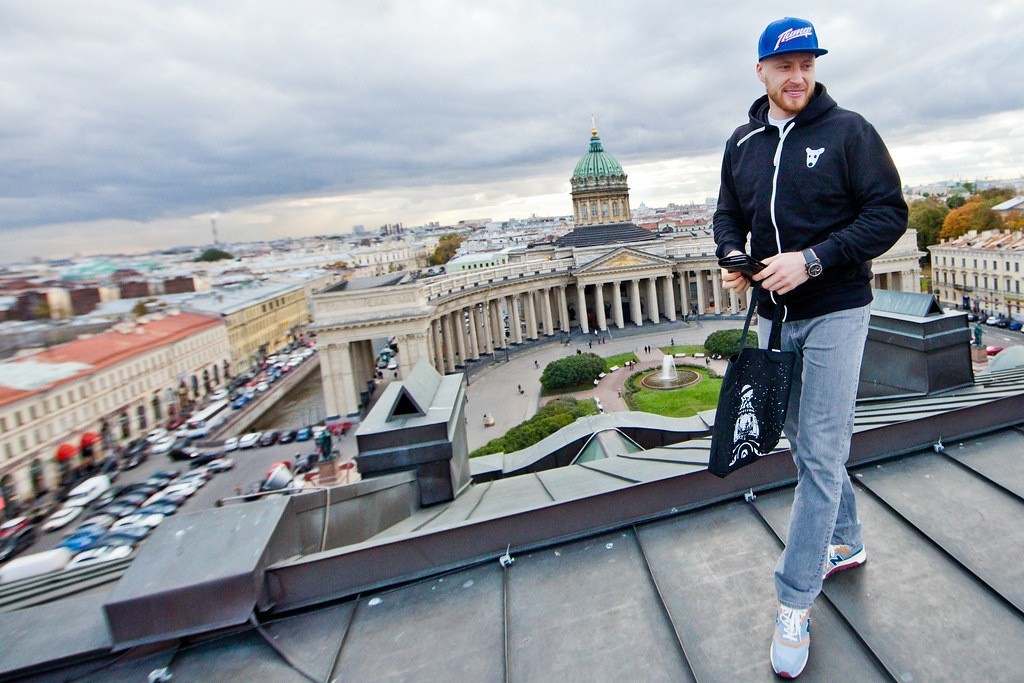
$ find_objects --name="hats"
[758,17,828,59]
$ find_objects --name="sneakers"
[770,597,810,680]
[822,539,865,581]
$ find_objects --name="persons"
[711,17,909,680]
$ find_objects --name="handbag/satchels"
[708,281,798,479]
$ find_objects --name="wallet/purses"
[718,255,769,281]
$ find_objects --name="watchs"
[801,247,822,279]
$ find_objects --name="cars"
[1,329,399,576]
[967,312,1024,357]
